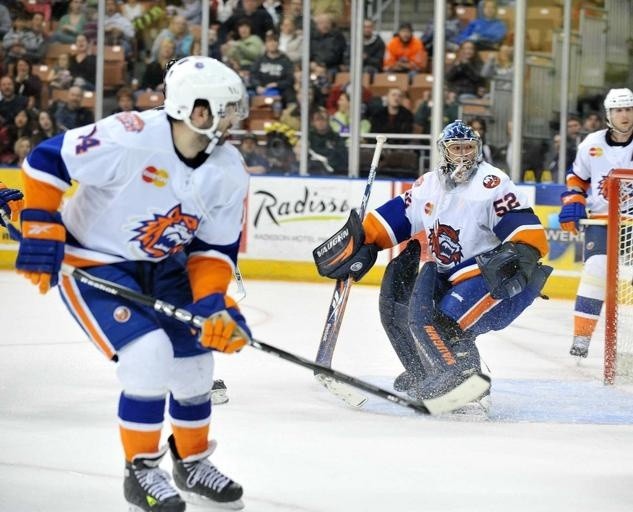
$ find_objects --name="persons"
[557,86,633,358]
[522,94,607,184]
[12,55,252,511]
[313,122,557,404]
[1,0,506,179]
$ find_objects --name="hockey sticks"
[314,135,387,409]
[61,264,491,414]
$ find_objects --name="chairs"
[0,0,601,174]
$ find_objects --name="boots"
[124,443,186,512]
[168,434,242,502]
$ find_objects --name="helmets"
[163,56,250,145]
[437,120,483,183]
[603,88,633,135]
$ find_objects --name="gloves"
[187,250,252,354]
[15,208,66,294]
[559,194,586,235]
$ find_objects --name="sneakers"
[572,334,589,355]
